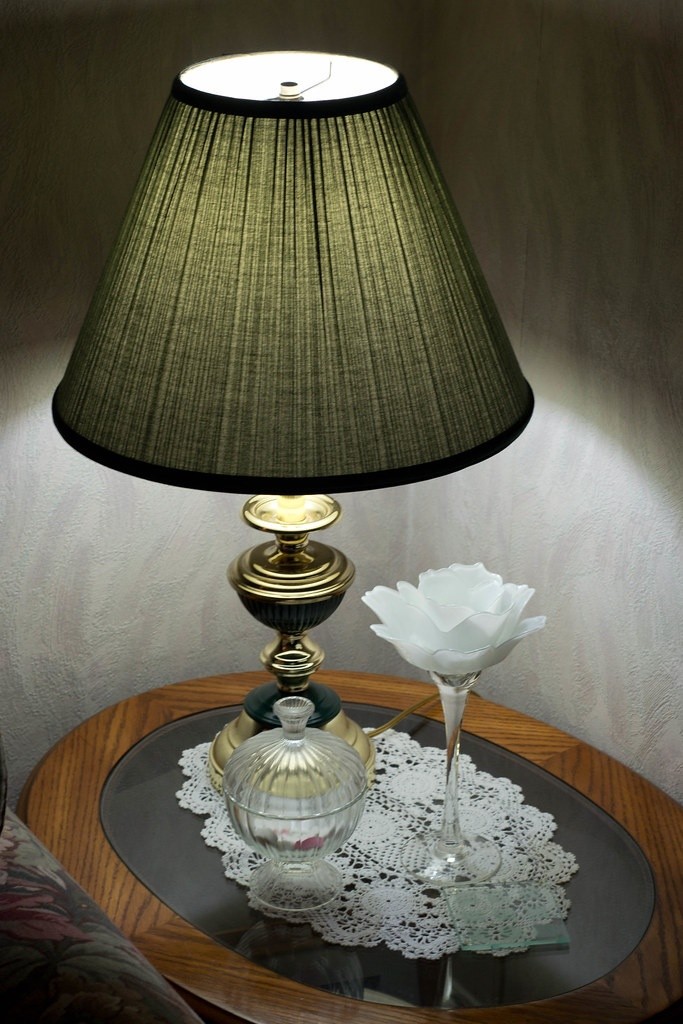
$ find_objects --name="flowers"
[360,562,548,674]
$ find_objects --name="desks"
[18,670,683,1024]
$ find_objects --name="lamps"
[52,51,536,800]
